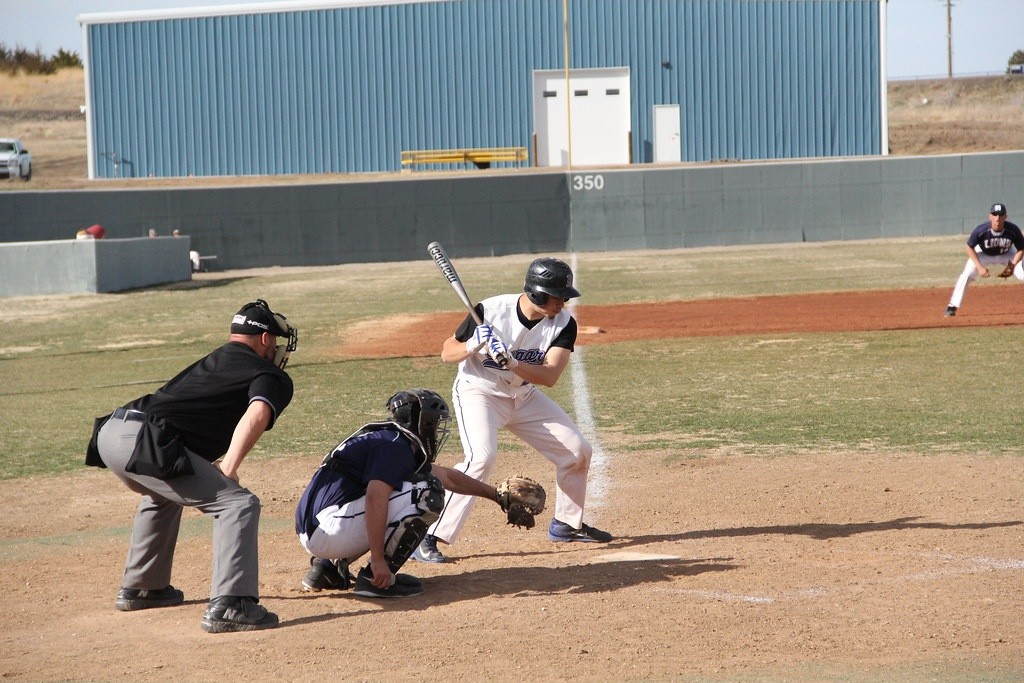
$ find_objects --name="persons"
[944,203,1024,318]
[294,388,546,598]
[410,258,613,564]
[85,299,299,634]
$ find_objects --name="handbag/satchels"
[85,411,114,469]
[125,415,195,480]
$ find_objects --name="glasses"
[992,213,1004,216]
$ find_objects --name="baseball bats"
[427,242,508,366]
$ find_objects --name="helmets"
[523,257,581,306]
[386,392,420,430]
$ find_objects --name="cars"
[0,137,31,182]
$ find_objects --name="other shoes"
[945,307,955,316]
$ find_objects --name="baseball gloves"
[997,260,1015,277]
[497,474,546,530]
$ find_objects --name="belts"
[113,408,146,422]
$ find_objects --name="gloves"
[488,339,518,370]
[466,325,494,354]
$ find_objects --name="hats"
[231,303,289,338]
[991,204,1005,213]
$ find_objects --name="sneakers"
[115,586,184,610]
[549,517,611,542]
[353,567,424,597]
[201,596,278,632]
[408,534,448,563]
[302,558,357,590]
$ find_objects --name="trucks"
[1010,63,1024,74]
